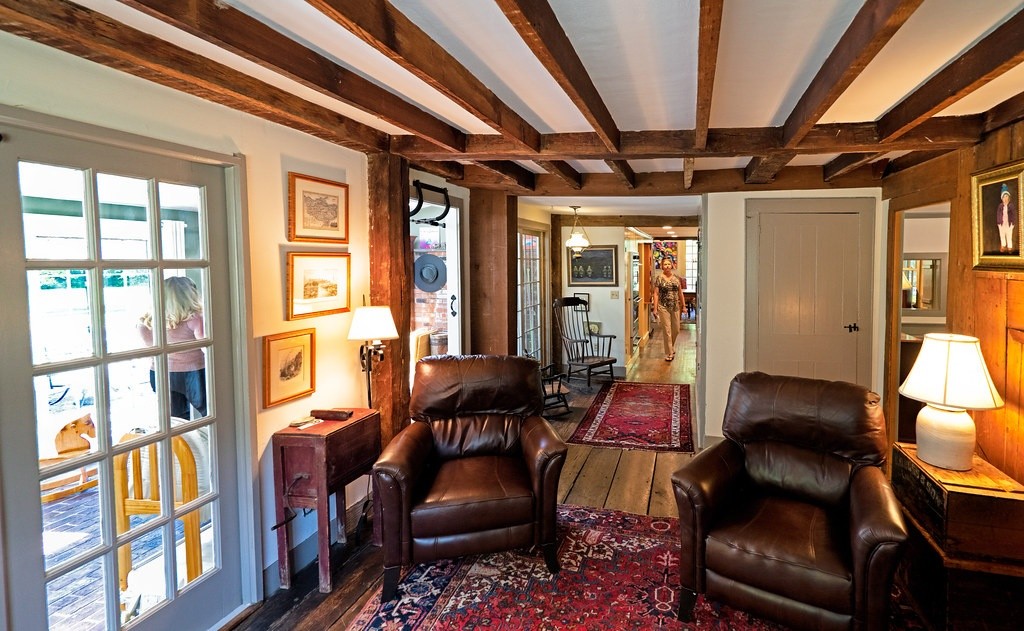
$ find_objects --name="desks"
[679,288,696,319]
[893,446,1024,631]
[272,408,381,593]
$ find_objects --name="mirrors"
[902,252,949,317]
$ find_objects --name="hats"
[414,254,447,291]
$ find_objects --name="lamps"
[566,206,593,258]
[899,333,1005,472]
[902,271,912,289]
[348,305,399,409]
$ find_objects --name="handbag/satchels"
[55,415,95,453]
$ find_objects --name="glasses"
[662,257,670,260]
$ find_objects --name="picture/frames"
[262,328,315,409]
[286,253,351,321]
[567,245,619,287]
[970,158,1024,271]
[574,293,589,311]
[288,171,349,244]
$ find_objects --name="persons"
[138,277,206,421]
[653,259,688,361]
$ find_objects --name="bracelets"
[683,305,686,307]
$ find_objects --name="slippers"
[672,351,676,354]
[666,355,674,361]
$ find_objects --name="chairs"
[523,349,573,416]
[553,296,617,386]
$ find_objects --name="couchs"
[671,372,907,631]
[372,355,568,605]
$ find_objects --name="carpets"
[345,503,773,631]
[566,381,695,453]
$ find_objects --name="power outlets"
[610,291,619,299]
